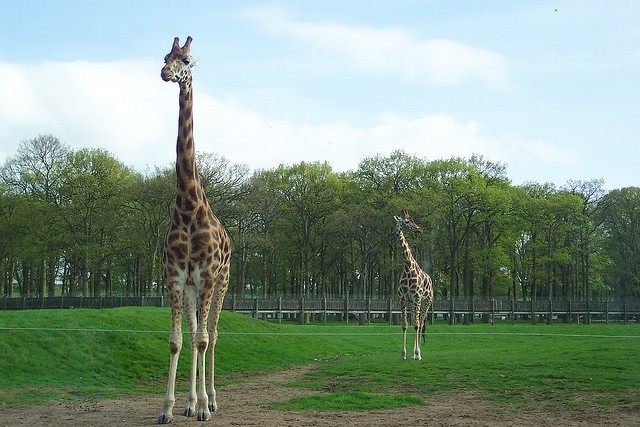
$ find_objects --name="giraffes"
[157,36,232,424]
[392,210,433,361]
